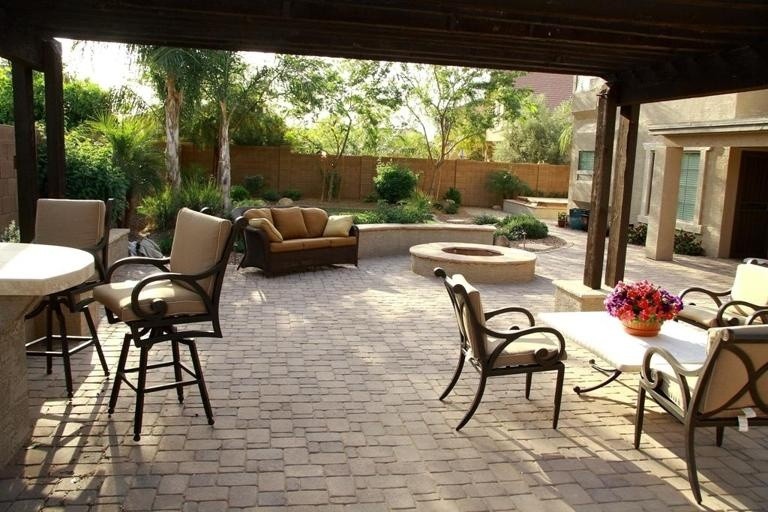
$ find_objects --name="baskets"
[621,317,661,337]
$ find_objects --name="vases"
[623,318,665,338]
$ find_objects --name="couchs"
[231,204,361,277]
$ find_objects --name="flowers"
[603,280,682,321]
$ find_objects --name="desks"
[545,312,717,419]
[0,242,94,467]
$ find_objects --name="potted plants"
[558,211,566,226]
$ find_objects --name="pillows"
[322,214,354,236]
[248,217,284,243]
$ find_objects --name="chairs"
[27,198,116,397]
[633,326,768,502]
[672,258,768,329]
[93,205,245,442]
[433,267,573,431]
